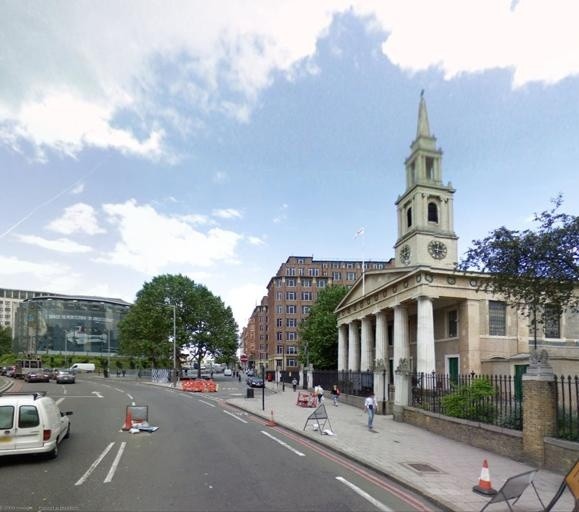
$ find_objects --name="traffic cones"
[122,411,134,432]
[265,410,276,428]
[472,458,499,496]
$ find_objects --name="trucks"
[69,363,96,374]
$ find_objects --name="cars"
[245,369,257,377]
[0,365,77,384]
[247,377,266,388]
[181,363,232,384]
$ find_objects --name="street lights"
[168,304,177,387]
[302,340,309,367]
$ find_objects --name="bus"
[14,353,42,379]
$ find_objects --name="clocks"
[399,244,411,263]
[429,239,448,258]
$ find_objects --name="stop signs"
[240,354,248,363]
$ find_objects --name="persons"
[363,392,380,429]
[316,384,325,403]
[292,377,298,392]
[331,383,340,407]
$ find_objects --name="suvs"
[0,391,72,459]
